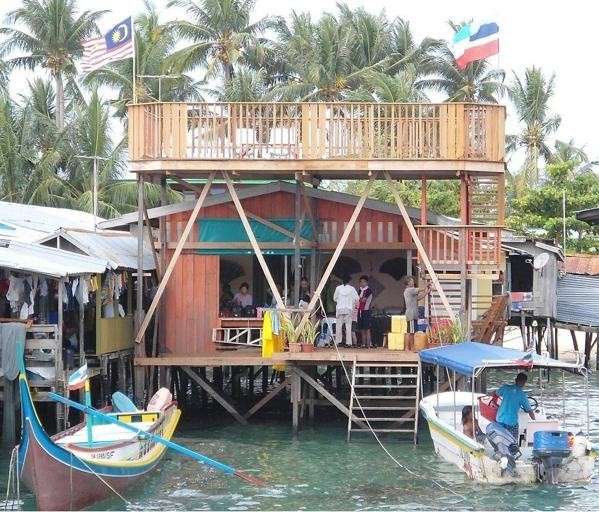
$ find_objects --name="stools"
[321,318,337,339]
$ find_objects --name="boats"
[10,337,183,510]
[415,342,596,489]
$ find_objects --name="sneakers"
[358,344,373,349]
[337,343,351,348]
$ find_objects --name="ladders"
[212,328,262,348]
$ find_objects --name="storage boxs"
[533,430,570,450]
[391,315,407,333]
[388,332,405,351]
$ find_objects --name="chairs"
[232,123,329,156]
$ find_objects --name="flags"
[78,16,134,76]
[452,14,501,69]
[516,349,534,368]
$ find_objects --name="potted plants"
[299,317,321,353]
[280,313,301,353]
[427,323,440,348]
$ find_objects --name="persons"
[220,283,235,312]
[332,273,360,347]
[487,372,537,477]
[460,404,485,440]
[402,275,432,354]
[357,275,373,348]
[267,276,311,309]
[231,281,252,314]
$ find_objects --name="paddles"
[45,392,271,486]
